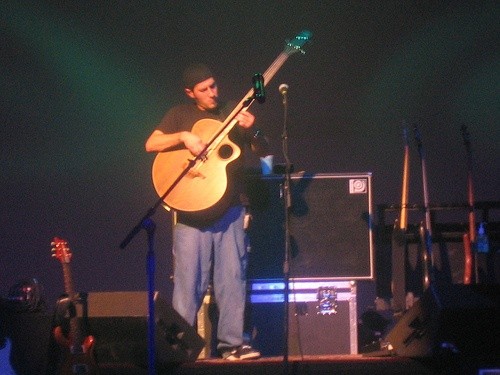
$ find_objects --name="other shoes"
[224,344,262,360]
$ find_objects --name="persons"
[142,61,273,360]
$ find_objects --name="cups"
[260,155,273,175]
[317,287,338,315]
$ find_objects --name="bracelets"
[250,128,264,141]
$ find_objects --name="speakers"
[378,282,500,362]
[70,291,207,367]
[243,172,374,283]
[0,293,77,375]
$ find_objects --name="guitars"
[151,26,313,220]
[48,236,98,375]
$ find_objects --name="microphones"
[252,72,265,103]
[278,84,289,96]
